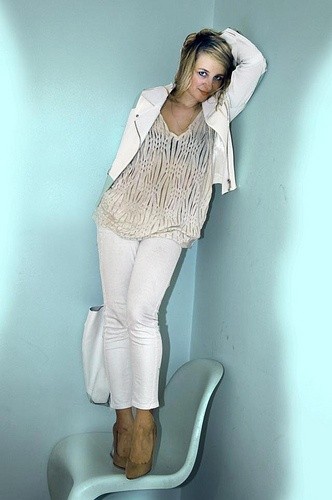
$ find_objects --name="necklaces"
[170,99,198,133]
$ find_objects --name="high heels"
[110,423,131,469]
[125,421,156,479]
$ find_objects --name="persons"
[90,27,269,480]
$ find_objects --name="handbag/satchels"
[82,304,111,407]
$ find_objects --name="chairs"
[46,359,224,500]
